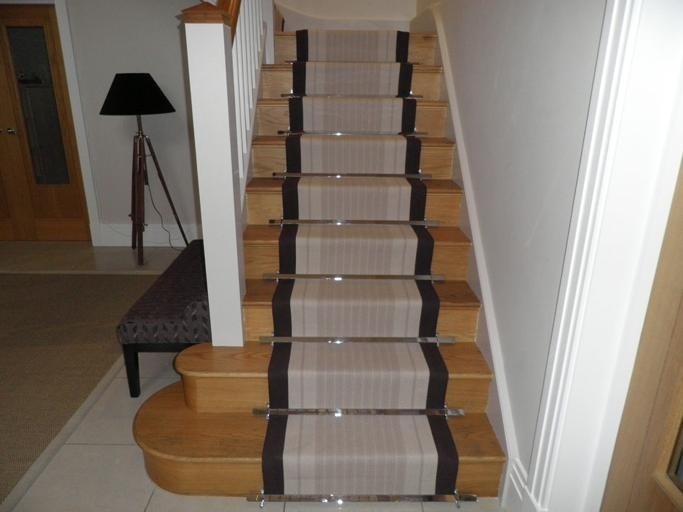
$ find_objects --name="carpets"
[259,23,459,495]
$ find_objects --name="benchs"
[114,237,213,400]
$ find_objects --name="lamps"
[97,72,190,269]
